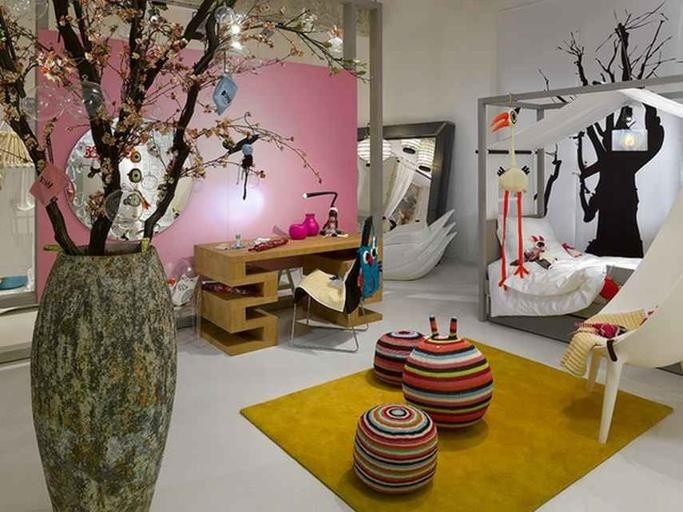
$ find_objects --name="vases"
[28,246,178,512]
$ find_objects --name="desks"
[188,233,381,356]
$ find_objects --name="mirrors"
[63,117,195,243]
[357,120,455,267]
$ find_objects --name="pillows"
[495,211,572,261]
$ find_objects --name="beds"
[476,74,681,377]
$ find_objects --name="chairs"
[288,214,374,353]
[575,186,682,444]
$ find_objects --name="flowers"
[0,0,375,258]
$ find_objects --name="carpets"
[240,338,674,512]
